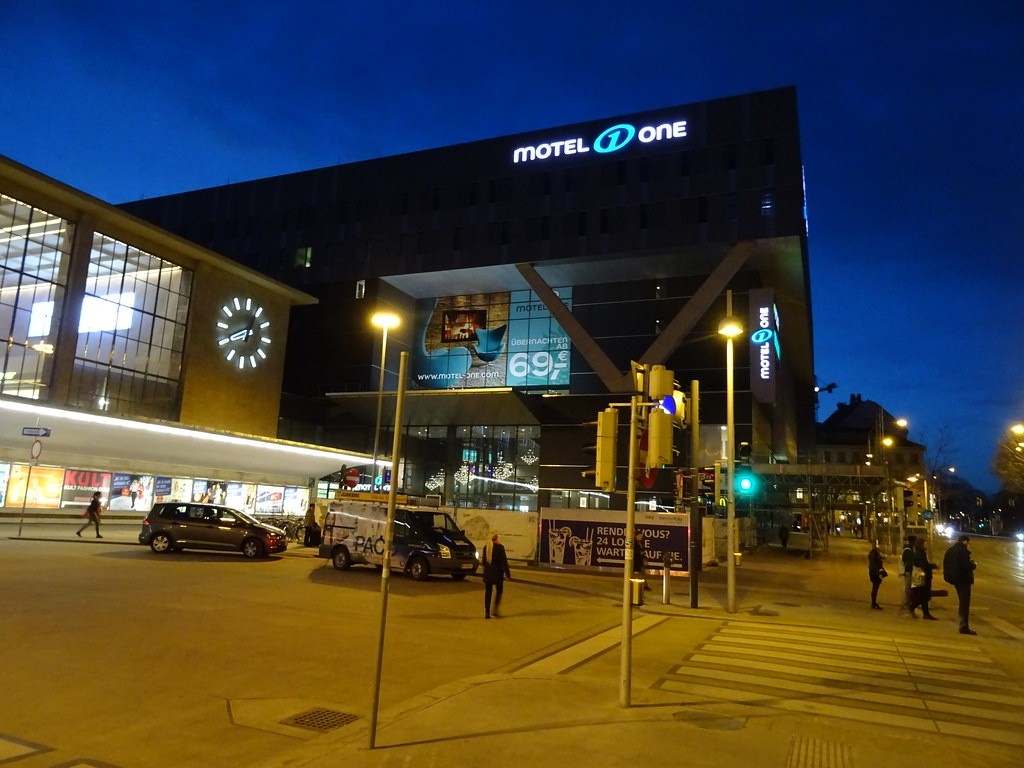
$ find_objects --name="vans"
[317,489,481,582]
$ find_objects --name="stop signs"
[344,468,360,488]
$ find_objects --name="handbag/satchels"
[83,509,89,518]
[910,567,926,588]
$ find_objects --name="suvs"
[138,502,290,559]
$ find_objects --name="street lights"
[370,311,401,493]
[868,416,908,465]
[717,288,744,615]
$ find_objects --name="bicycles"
[251,513,306,543]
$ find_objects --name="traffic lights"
[903,489,914,508]
[736,475,755,494]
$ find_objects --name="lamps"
[454,429,476,485]
[425,469,446,491]
[520,426,538,464]
[523,475,540,493]
[491,427,514,480]
[473,428,490,473]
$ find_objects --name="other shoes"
[871,604,882,610]
[97,535,103,538]
[924,614,938,620]
[959,627,977,635]
[484,615,491,620]
[909,607,919,619]
[491,613,502,618]
[76,532,81,537]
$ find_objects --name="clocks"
[217,296,273,370]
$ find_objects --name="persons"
[208,483,222,504]
[903,535,938,620]
[634,531,652,591]
[111,476,147,509]
[834,522,842,537]
[304,503,316,547]
[76,491,103,539]
[778,522,791,547]
[952,536,979,636]
[483,526,510,620]
[853,521,860,535]
[869,538,888,610]
[321,509,349,545]
